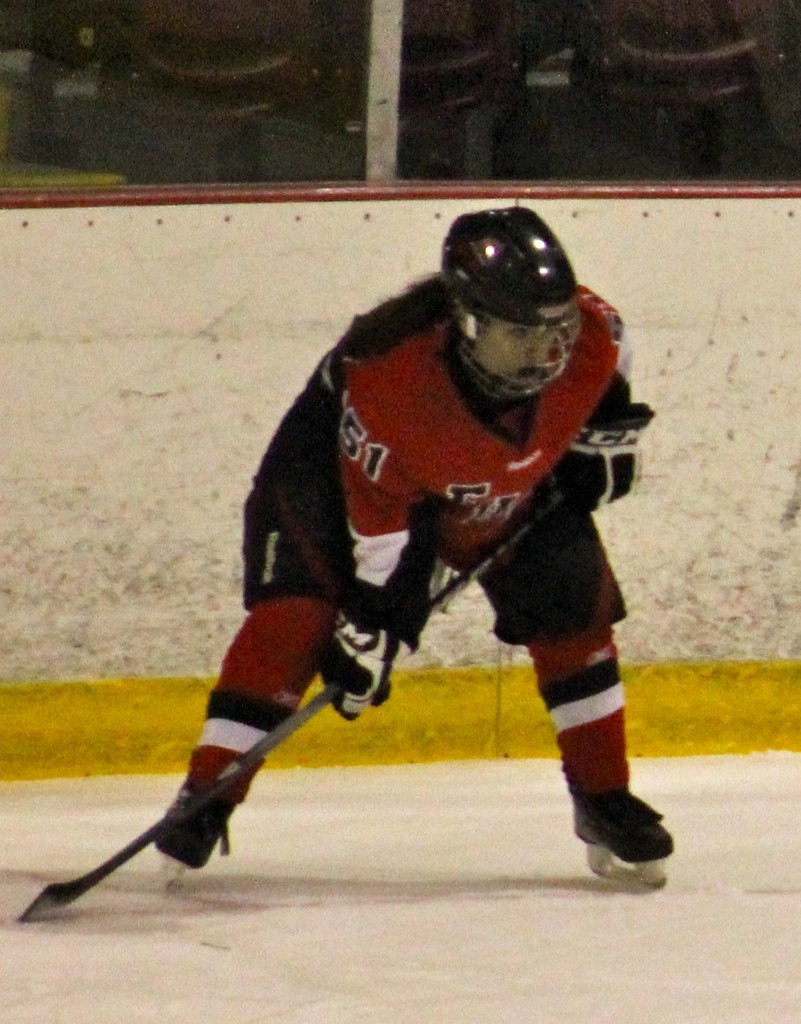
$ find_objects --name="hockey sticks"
[18,490,565,920]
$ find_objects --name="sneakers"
[155,782,232,887]
[574,788,675,884]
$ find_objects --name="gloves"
[322,577,402,721]
[551,404,656,515]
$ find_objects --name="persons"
[151,208,675,896]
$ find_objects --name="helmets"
[443,207,579,402]
